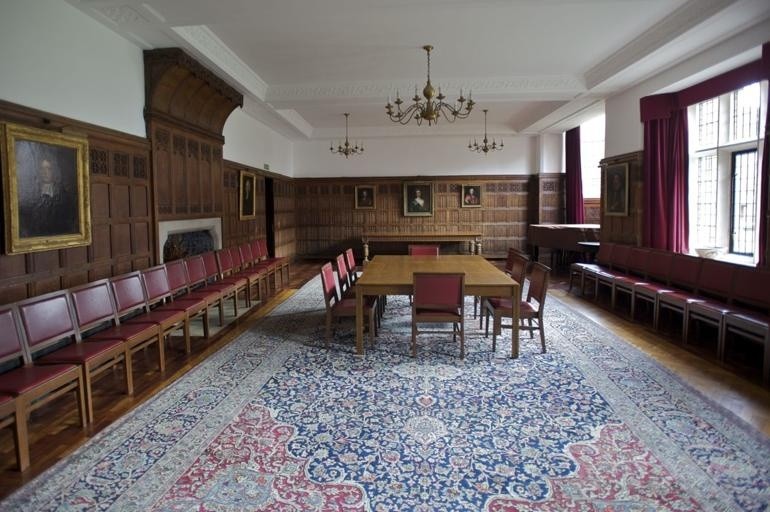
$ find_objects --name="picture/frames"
[0,122,92,255]
[604,164,629,218]
[462,184,483,207]
[239,169,256,221]
[355,185,376,210]
[403,181,434,217]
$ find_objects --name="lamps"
[385,47,476,127]
[468,109,504,154]
[330,113,364,160]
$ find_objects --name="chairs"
[15,239,292,423]
[567,242,770,391]
[321,245,551,360]
[0,391,31,471]
[0,304,88,469]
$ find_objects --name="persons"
[464,188,480,205]
[608,172,625,212]
[409,189,430,212]
[242,180,253,216]
[21,157,80,236]
[360,190,372,206]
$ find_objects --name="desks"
[529,224,601,260]
[361,232,483,261]
